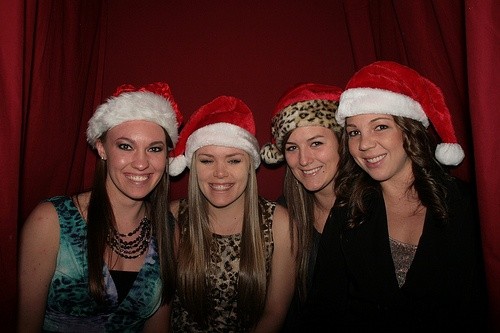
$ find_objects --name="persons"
[20,82,183,333]
[167,95,298,333]
[301,61,488,333]
[260,84,350,274]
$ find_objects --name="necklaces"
[108,204,153,259]
